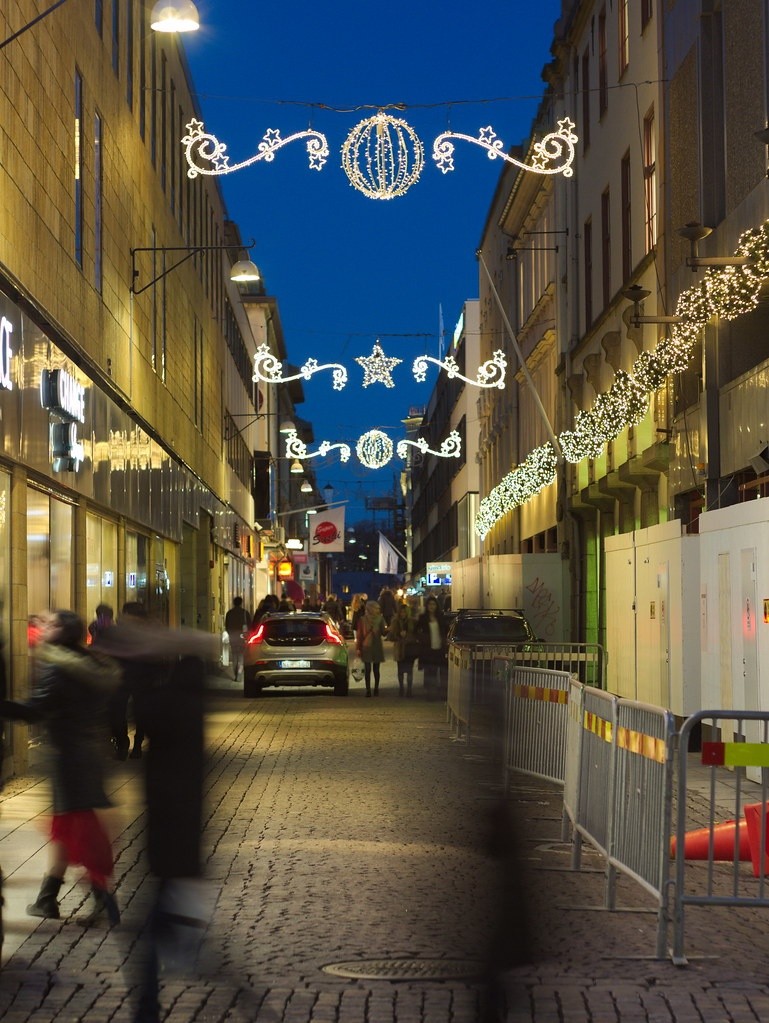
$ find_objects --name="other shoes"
[366,690,371,697]
[374,689,379,696]
[235,673,241,682]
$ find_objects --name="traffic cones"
[670,801,769,877]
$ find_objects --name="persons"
[356,601,389,697]
[378,585,396,641]
[252,593,296,629]
[89,603,145,760]
[5,609,122,931]
[225,597,252,682]
[391,605,417,696]
[351,593,368,643]
[416,596,448,702]
[303,593,346,626]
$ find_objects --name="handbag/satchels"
[242,623,248,633]
[351,653,365,681]
[393,639,405,662]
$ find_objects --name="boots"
[129,735,144,758]
[26,877,64,919]
[75,886,120,927]
[119,736,129,759]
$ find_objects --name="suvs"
[445,608,539,675]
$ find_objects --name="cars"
[244,611,349,696]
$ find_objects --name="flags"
[379,535,398,574]
